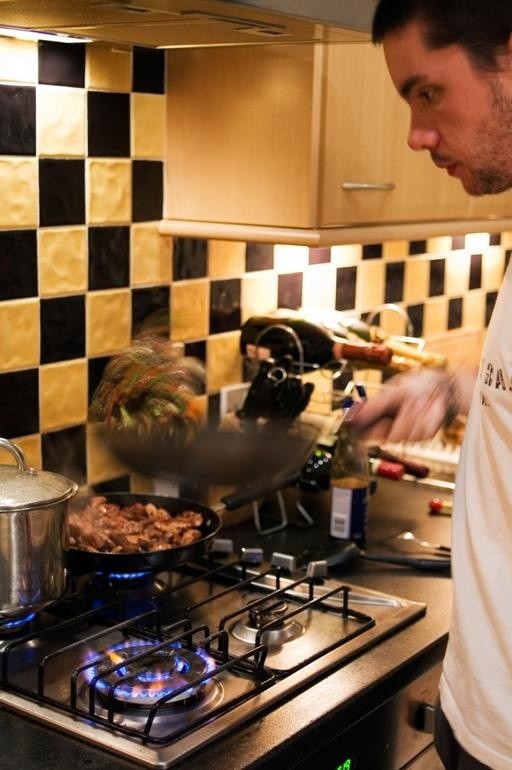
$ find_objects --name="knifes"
[237,360,317,432]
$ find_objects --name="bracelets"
[423,350,461,435]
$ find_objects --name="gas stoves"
[0,537,444,770]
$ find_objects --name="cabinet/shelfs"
[159,0,512,248]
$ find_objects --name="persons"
[338,0,511,768]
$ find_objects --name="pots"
[1,437,80,619]
[64,472,305,575]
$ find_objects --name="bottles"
[305,440,431,487]
[331,418,368,550]
[239,305,449,378]
